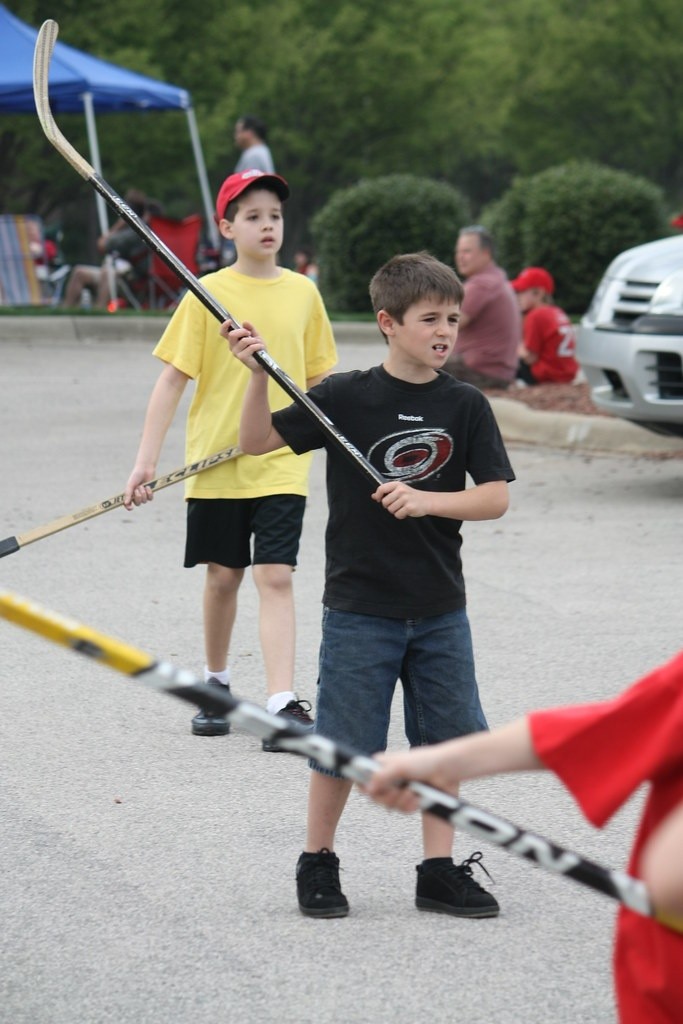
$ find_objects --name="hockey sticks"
[28,11,399,491]
[0,592,683,932]
[0,444,242,560]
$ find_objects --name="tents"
[0,2,220,312]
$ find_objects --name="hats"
[510,267,552,294]
[217,168,291,220]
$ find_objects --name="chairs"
[113,215,204,312]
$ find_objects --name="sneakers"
[192,678,231,735]
[415,852,500,918]
[263,693,316,754]
[295,848,349,919]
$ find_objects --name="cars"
[567,234,683,435]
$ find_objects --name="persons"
[510,267,580,387]
[439,226,523,390]
[361,645,683,1023]
[294,255,318,288]
[234,114,274,173]
[123,169,339,753]
[62,191,160,307]
[219,251,516,918]
